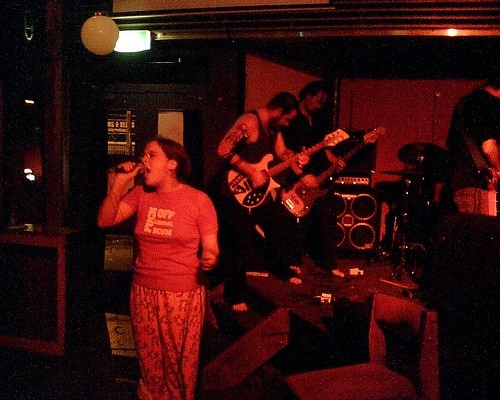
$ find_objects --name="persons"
[274,80,345,277]
[446,70,500,216]
[216,90,310,311]
[98,137,219,400]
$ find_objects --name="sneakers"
[307,255,336,273]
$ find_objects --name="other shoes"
[223,290,257,308]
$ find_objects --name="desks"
[0,224,92,356]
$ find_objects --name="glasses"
[140,151,167,159]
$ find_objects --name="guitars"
[279,127,387,217]
[227,129,351,207]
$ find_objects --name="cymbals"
[383,167,413,178]
[398,142,442,166]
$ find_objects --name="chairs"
[284,292,440,400]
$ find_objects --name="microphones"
[108,162,147,173]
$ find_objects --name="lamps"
[81,11,119,55]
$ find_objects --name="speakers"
[329,187,379,258]
